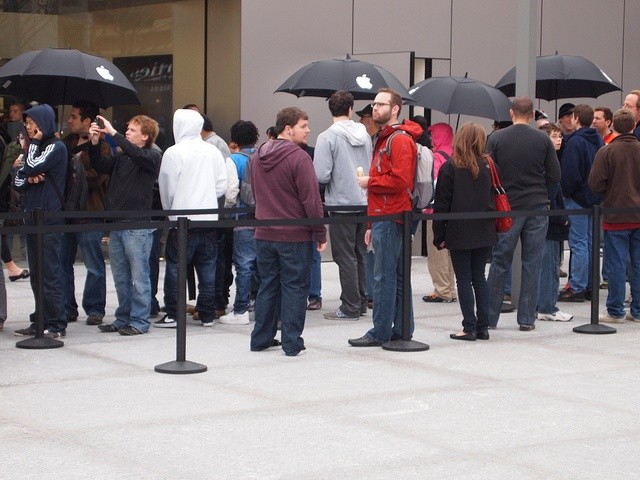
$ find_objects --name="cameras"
[93,116,102,130]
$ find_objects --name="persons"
[592,106,615,290]
[622,89,640,143]
[219,120,258,326]
[154,108,227,326]
[5,101,28,121]
[432,123,498,340]
[64,99,112,326]
[588,109,640,323]
[13,103,68,339]
[533,109,549,128]
[313,92,373,321]
[485,96,561,331]
[297,142,323,311]
[194,113,232,319]
[408,115,433,240]
[558,104,606,302]
[355,103,382,310]
[348,89,417,346]
[484,117,512,144]
[555,104,579,278]
[251,107,327,355]
[1,238,31,282]
[90,115,162,335]
[540,122,565,151]
[422,123,458,302]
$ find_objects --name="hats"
[559,103,576,118]
[355,104,373,116]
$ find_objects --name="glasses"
[371,101,390,107]
[550,134,563,139]
[22,120,35,127]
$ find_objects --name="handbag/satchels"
[486,155,513,232]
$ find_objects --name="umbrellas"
[402,72,513,133]
[0,49,142,136]
[494,51,622,125]
[273,54,416,102]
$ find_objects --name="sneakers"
[97,324,118,332]
[598,309,628,324]
[189,307,224,320]
[153,314,177,328]
[536,310,573,321]
[119,326,143,334]
[33,328,66,339]
[248,306,255,321]
[626,312,640,322]
[422,294,457,303]
[558,287,593,302]
[251,340,282,351]
[218,307,256,324]
[323,308,367,323]
[13,323,46,336]
[520,324,535,331]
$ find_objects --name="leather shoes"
[86,315,102,325]
[477,330,489,339]
[307,297,322,310]
[9,269,30,282]
[450,330,477,341]
[500,303,514,313]
[348,331,412,346]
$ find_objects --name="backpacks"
[38,137,88,224]
[376,130,434,212]
[232,147,256,208]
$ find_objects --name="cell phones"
[33,129,39,134]
